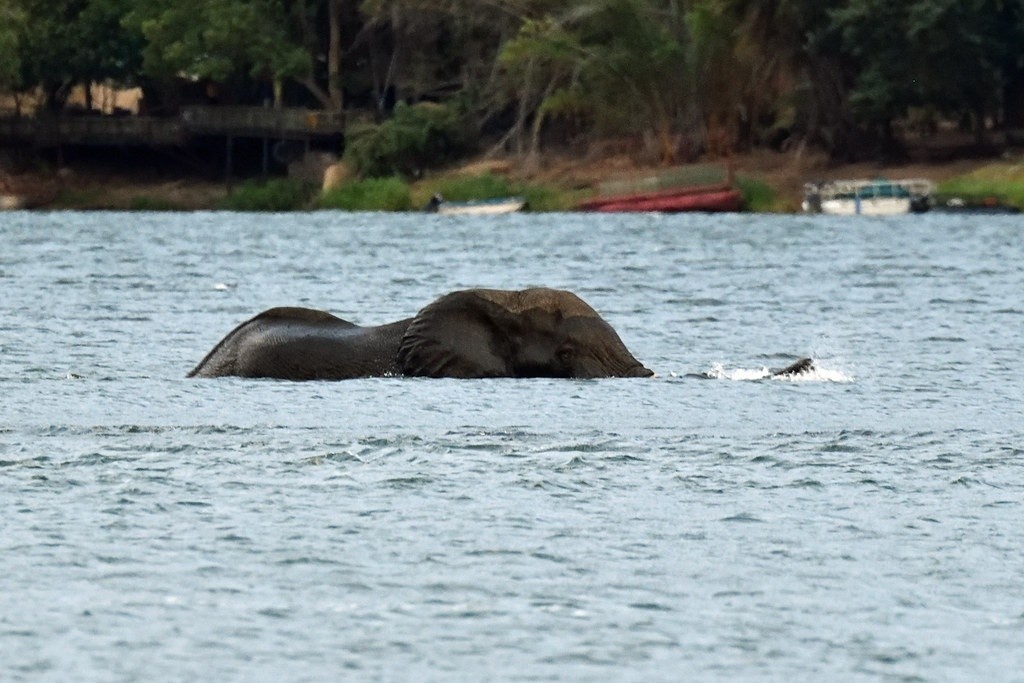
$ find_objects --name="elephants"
[184,286,813,381]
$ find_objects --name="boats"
[427,193,526,215]
[573,161,746,214]
[801,177,935,218]
